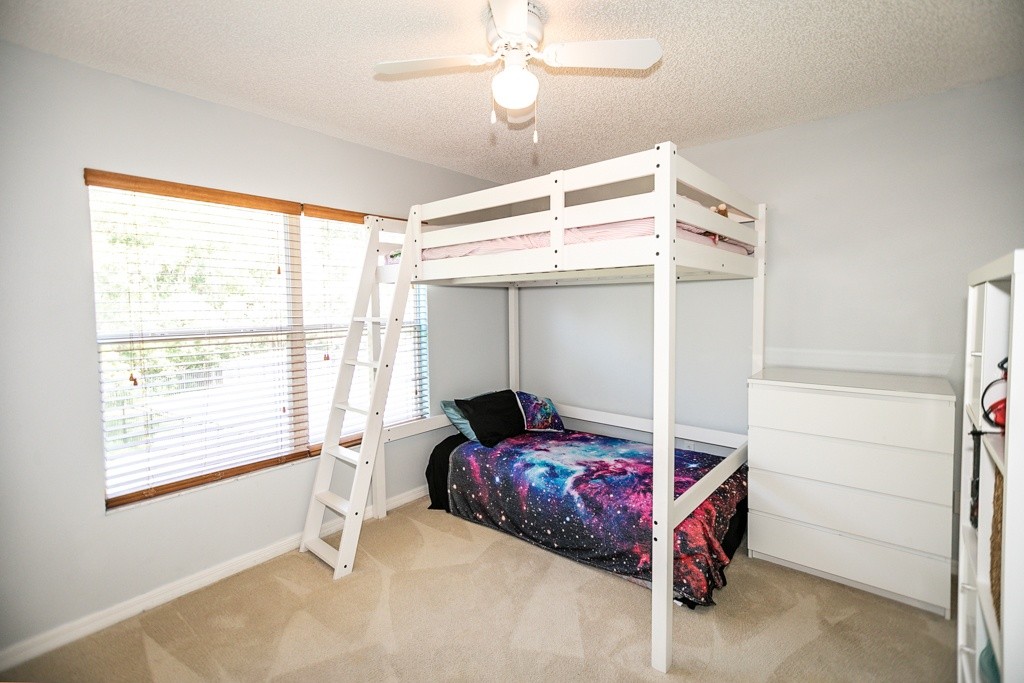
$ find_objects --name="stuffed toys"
[710,204,728,218]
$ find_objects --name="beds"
[378,142,766,286]
[425,388,748,610]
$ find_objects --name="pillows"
[454,389,530,447]
[439,401,480,445]
[514,390,567,434]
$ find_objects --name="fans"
[373,0,664,146]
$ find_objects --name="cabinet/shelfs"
[943,249,1024,683]
[744,368,958,620]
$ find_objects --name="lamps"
[490,49,542,142]
